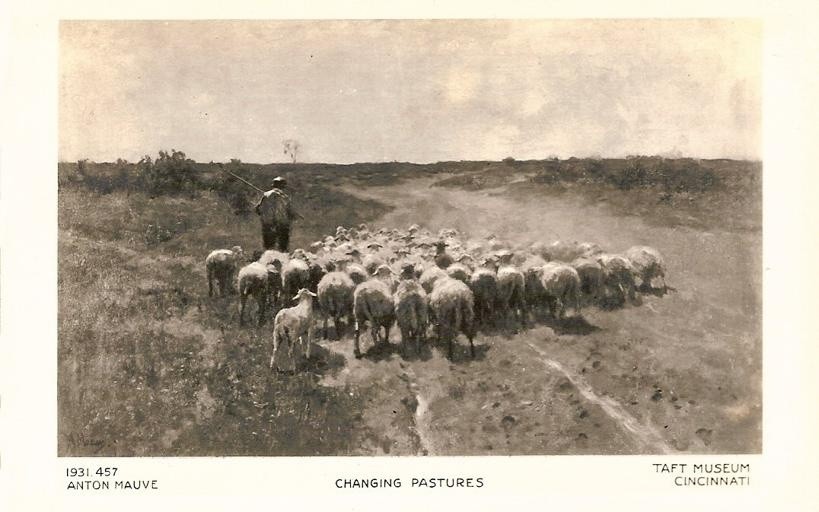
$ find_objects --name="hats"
[270,176,289,189]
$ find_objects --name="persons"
[254,177,300,252]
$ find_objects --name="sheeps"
[625,244,668,294]
[269,287,318,378]
[205,245,243,299]
[236,222,636,362]
